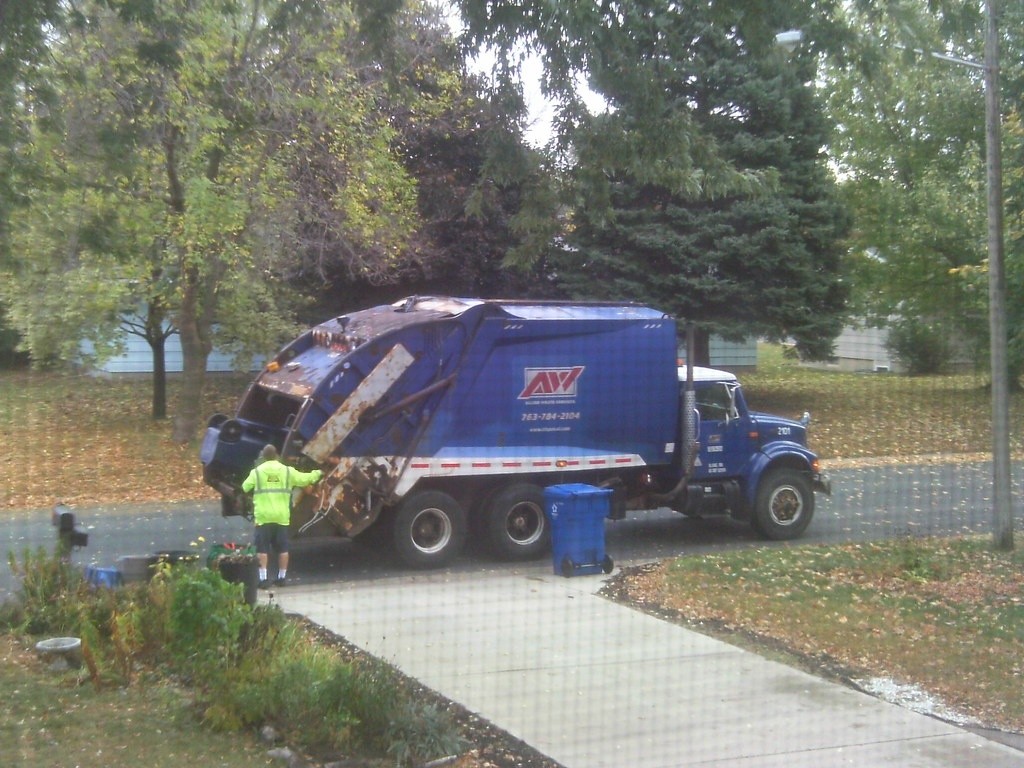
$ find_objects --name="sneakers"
[260,579,268,589]
[276,577,285,587]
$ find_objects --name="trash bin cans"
[154,549,200,574]
[219,555,262,610]
[542,483,615,579]
[207,543,258,575]
[200,414,266,472]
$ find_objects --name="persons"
[240,444,329,589]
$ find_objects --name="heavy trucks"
[199,297,833,570]
